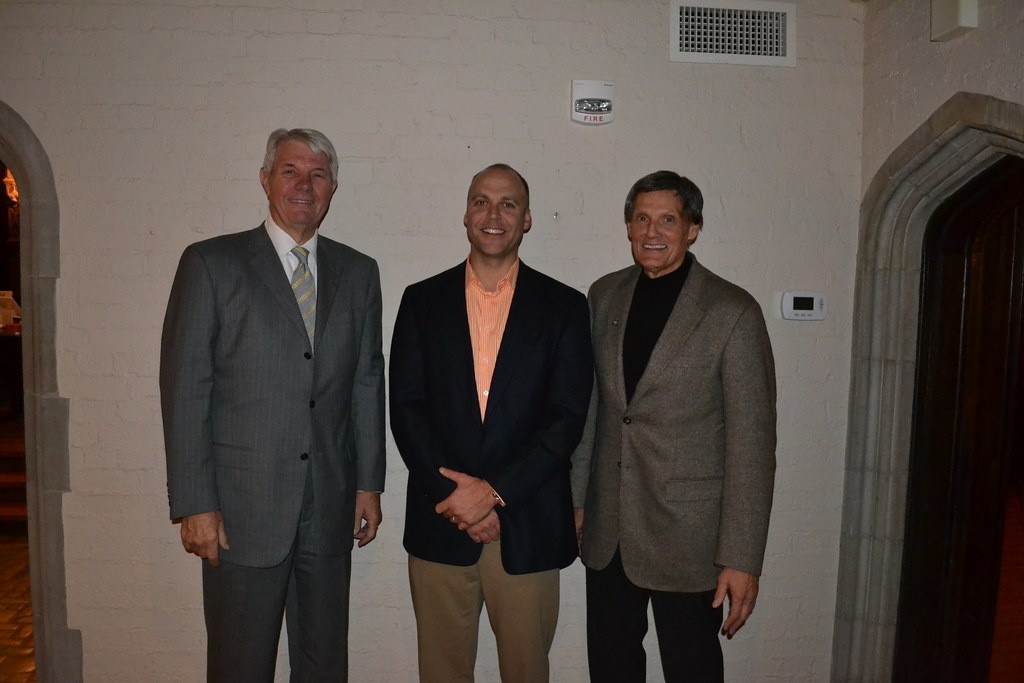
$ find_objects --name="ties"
[291,246,315,348]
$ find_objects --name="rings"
[452,516,456,523]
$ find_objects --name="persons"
[389,163,595,682]
[568,166,777,683]
[157,127,387,683]
